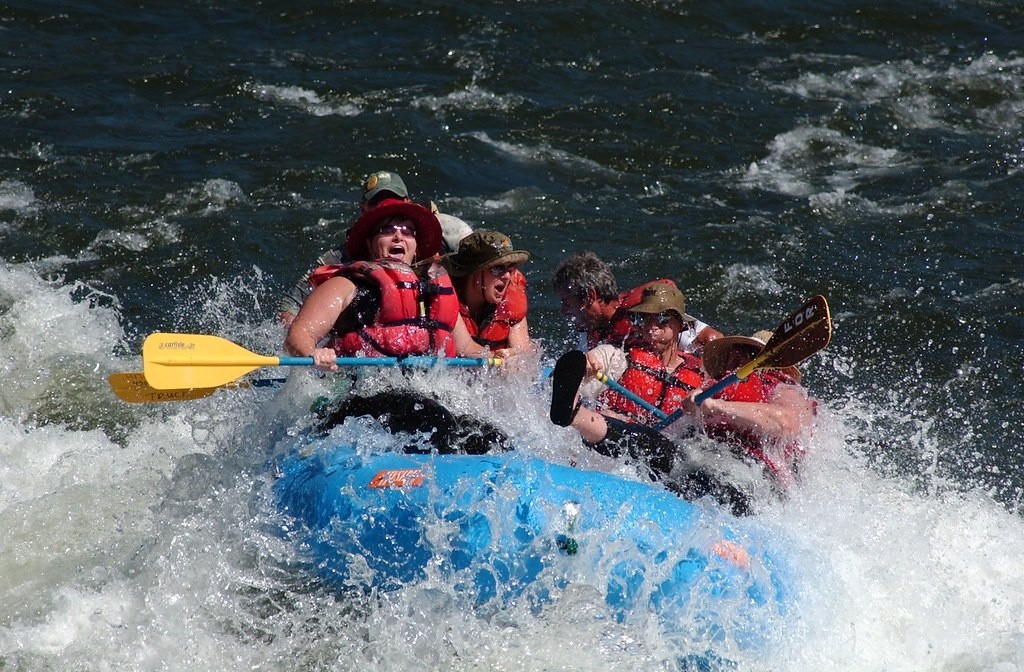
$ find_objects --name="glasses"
[731,343,760,360]
[635,311,681,327]
[481,263,517,277]
[371,223,417,243]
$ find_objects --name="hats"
[628,283,697,323]
[343,198,443,266]
[357,171,408,202]
[438,229,530,277]
[703,331,801,384]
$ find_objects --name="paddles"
[109,372,286,404]
[143,333,507,390]
[594,370,668,420]
[650,294,832,430]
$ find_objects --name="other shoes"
[550,350,587,427]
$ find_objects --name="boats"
[272,374,804,672]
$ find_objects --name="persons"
[551,251,817,517]
[279,171,532,376]
